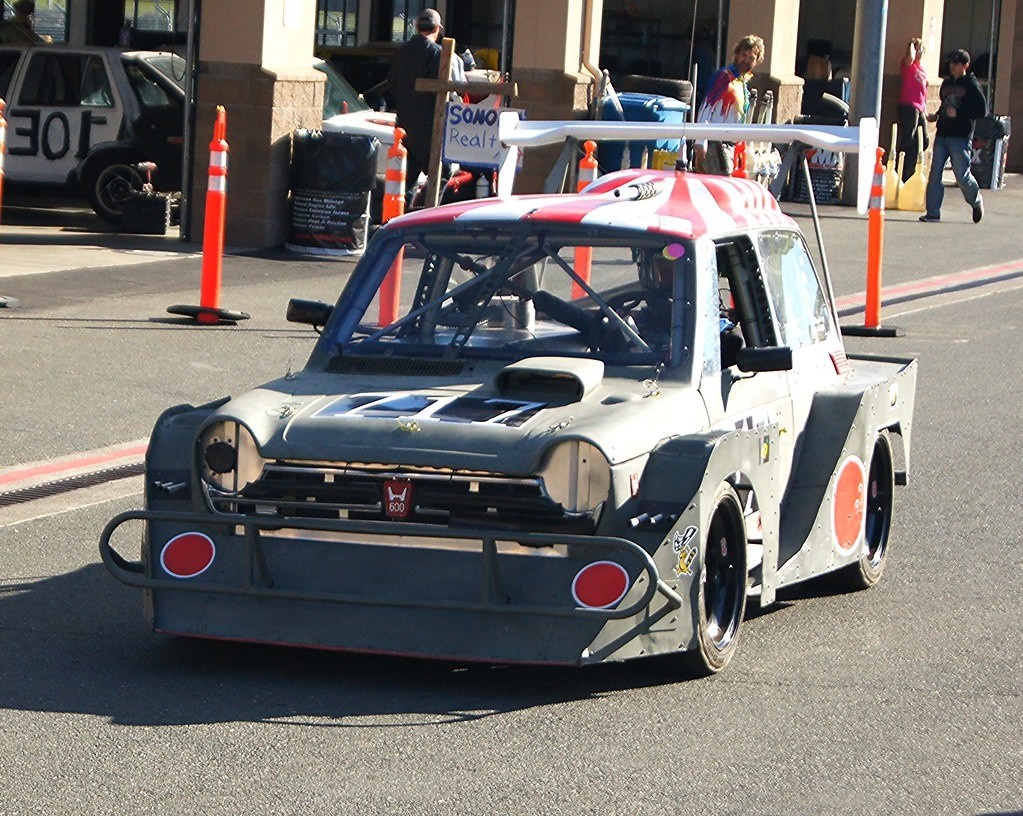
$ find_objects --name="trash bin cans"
[971,114,1011,188]
[285,129,379,254]
[601,91,691,174]
[794,115,848,202]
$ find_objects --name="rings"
[695,149,698,151]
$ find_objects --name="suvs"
[0,43,195,227]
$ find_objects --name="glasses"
[914,49,923,54]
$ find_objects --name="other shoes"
[919,215,940,222]
[972,198,983,223]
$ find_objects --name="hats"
[949,49,969,64]
[418,9,444,29]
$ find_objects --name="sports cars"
[98,170,918,672]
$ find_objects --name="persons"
[0,0,36,32]
[899,38,929,184]
[384,9,476,204]
[116,18,137,50]
[693,35,764,178]
[919,50,987,224]
